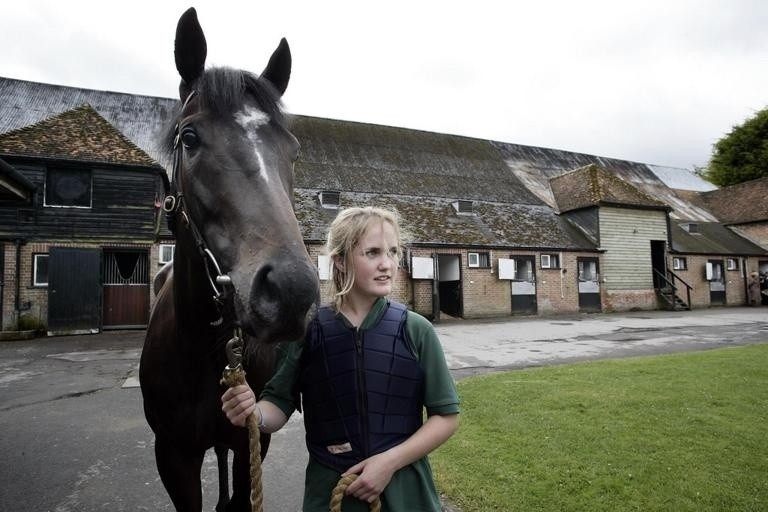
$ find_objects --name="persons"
[748,271,768,306]
[220,205,463,512]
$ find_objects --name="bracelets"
[257,403,266,428]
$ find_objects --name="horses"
[140,6,321,512]
